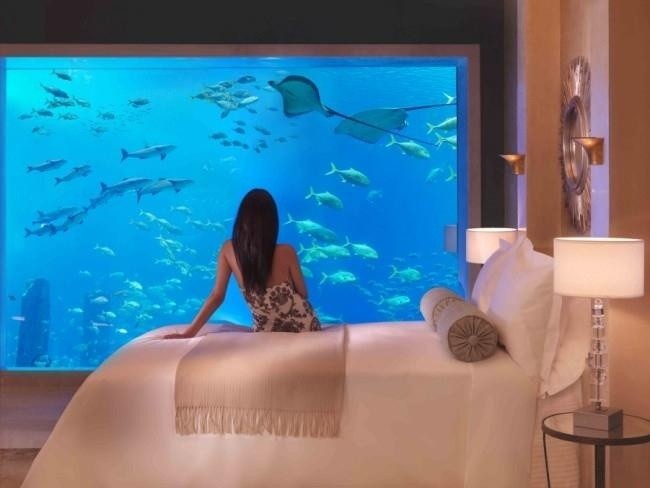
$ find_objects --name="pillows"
[437,299,499,361]
[468,234,567,379]
[420,287,465,331]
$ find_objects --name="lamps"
[464,227,525,268]
[553,237,647,431]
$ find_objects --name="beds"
[15,231,596,487]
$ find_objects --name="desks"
[542,410,649,486]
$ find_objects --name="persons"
[163,188,323,340]
